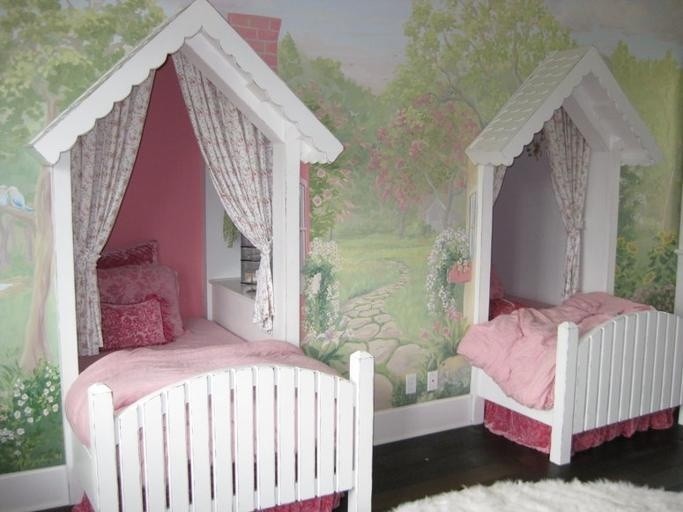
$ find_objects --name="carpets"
[388,476,683,512]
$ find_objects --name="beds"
[64,316,375,512]
[457,293,683,466]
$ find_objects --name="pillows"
[97,261,183,339]
[97,239,159,268]
[99,299,166,350]
